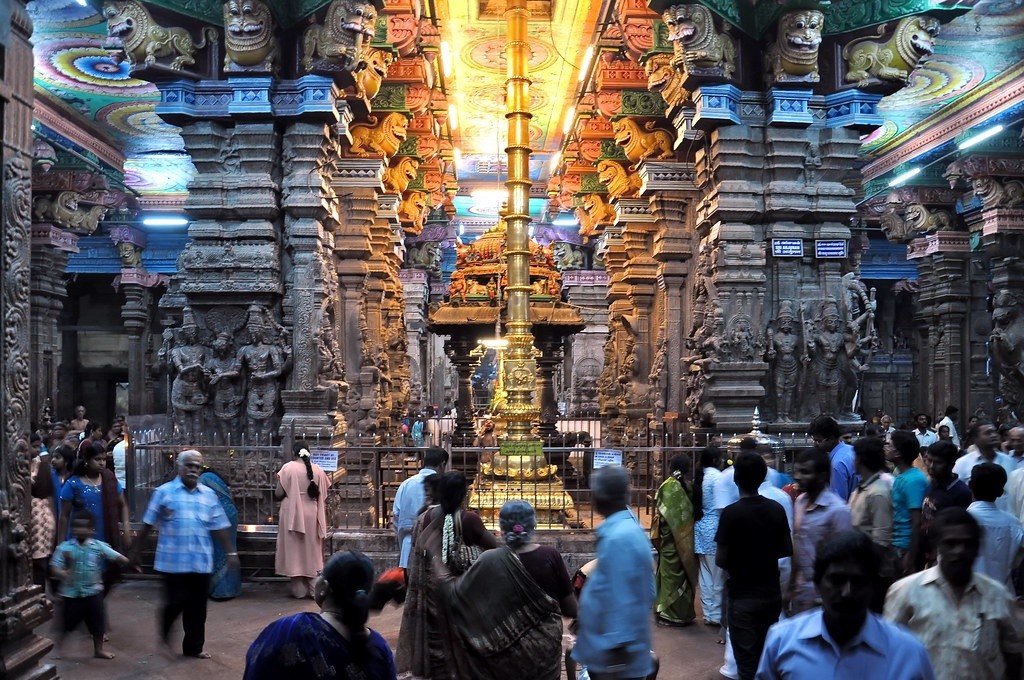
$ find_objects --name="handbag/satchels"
[449,509,484,576]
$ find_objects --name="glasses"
[316,569,333,593]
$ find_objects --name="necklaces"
[85,475,99,494]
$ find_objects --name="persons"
[766,300,872,423]
[601,295,714,408]
[171,305,282,460]
[199,465,242,598]
[50,511,143,659]
[58,443,132,641]
[419,499,579,680]
[394,470,497,680]
[473,419,497,464]
[129,450,235,659]
[401,401,458,447]
[650,405,1024,680]
[565,464,659,680]
[242,549,398,680]
[754,529,936,680]
[29,406,159,589]
[275,441,332,600]
[883,507,1024,680]
[392,445,450,584]
[317,311,376,379]
